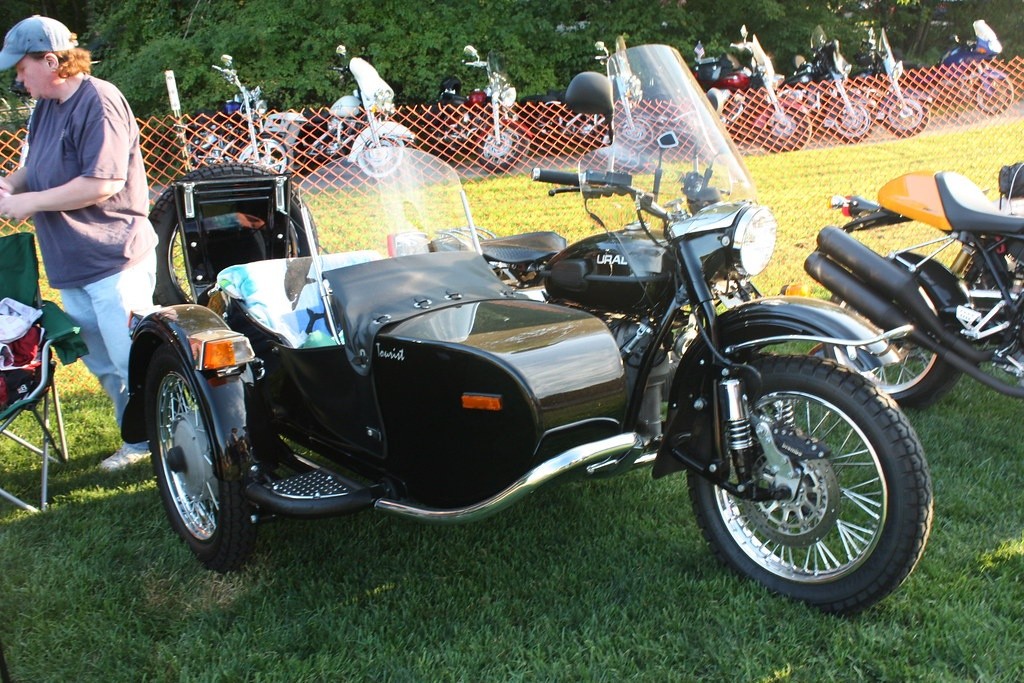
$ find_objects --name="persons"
[0,15,157,473]
[224,426,252,478]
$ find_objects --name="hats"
[0,15,79,72]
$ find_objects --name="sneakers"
[95,441,151,472]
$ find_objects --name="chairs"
[217,249,382,349]
[0,232,92,513]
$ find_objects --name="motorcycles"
[121,42,937,620]
[891,17,1017,118]
[283,42,417,181]
[777,158,1023,410]
[396,44,532,172]
[184,53,295,179]
[535,28,932,171]
